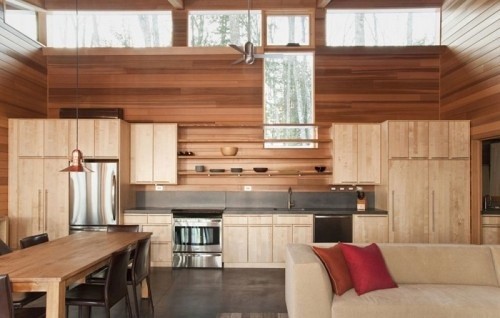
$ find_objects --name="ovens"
[172,218,223,268]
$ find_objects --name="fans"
[225,0,276,65]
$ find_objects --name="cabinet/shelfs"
[177,122,331,175]
[352,215,388,245]
[8,118,136,242]
[329,122,382,185]
[481,216,500,245]
[129,122,178,186]
[122,210,173,269]
[384,118,471,244]
[223,213,313,269]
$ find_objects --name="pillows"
[339,242,399,297]
[313,243,354,296]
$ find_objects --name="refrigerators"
[69,162,118,234]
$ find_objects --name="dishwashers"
[315,214,352,243]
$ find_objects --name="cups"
[196,166,204,172]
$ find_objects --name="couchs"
[284,242,500,318]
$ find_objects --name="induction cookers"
[171,209,223,218]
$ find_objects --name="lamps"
[59,0,93,173]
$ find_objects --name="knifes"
[356,191,365,200]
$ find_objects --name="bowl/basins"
[314,167,326,172]
[221,146,238,156]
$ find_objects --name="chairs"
[0,274,46,318]
[84,235,155,318]
[19,233,49,249]
[0,239,47,309]
[64,248,133,318]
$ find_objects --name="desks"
[0,228,153,318]
